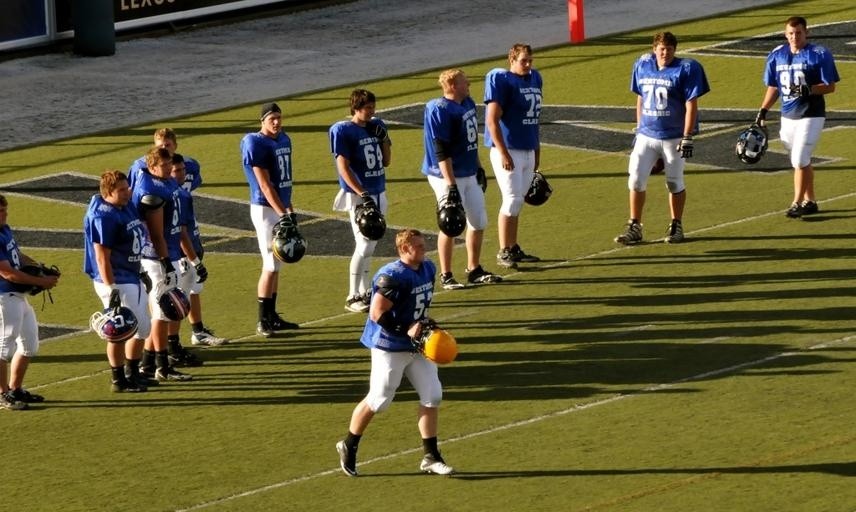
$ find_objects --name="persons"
[483,43,543,266]
[327,88,392,315]
[420,68,501,291]
[0,194,58,410]
[337,228,455,478]
[82,129,225,392]
[615,33,711,244]
[239,103,300,339]
[756,16,840,217]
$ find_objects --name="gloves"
[107,289,123,309]
[446,186,463,204]
[676,137,696,159]
[192,262,209,284]
[366,120,390,143]
[164,263,179,287]
[754,109,770,131]
[476,167,489,193]
[790,83,812,101]
[138,270,153,294]
[277,212,299,229]
[359,191,379,208]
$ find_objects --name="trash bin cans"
[73,0,116,58]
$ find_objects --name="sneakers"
[418,454,456,476]
[254,318,275,339]
[335,438,360,477]
[785,201,805,219]
[464,264,505,287]
[614,218,645,245]
[663,219,686,244]
[343,292,371,313]
[109,343,204,394]
[266,314,300,331]
[496,247,520,272]
[439,271,465,290]
[800,199,820,216]
[8,387,44,403]
[510,243,541,263]
[0,389,29,410]
[188,328,229,347]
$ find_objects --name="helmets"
[158,285,192,322]
[646,157,665,177]
[734,123,770,165]
[99,306,140,344]
[357,208,388,242]
[11,264,61,294]
[419,324,460,365]
[436,201,469,239]
[270,226,308,265]
[523,170,555,208]
